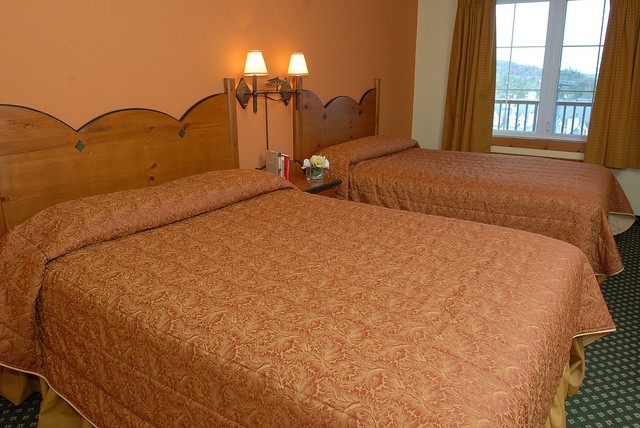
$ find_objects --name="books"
[280,157,285,178]
[265,149,282,177]
[280,153,290,181]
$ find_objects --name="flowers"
[309,153,330,177]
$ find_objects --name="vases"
[306,167,324,183]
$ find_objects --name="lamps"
[236,50,309,113]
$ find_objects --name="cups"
[306,165,311,180]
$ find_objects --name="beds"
[0,78,587,427]
[293,79,613,283]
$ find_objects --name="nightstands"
[289,161,343,200]
